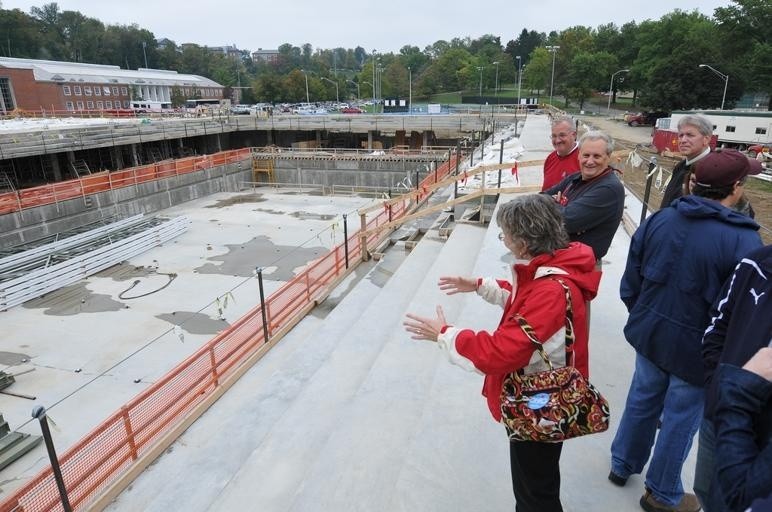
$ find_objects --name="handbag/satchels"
[499,278,610,444]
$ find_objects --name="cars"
[748,143,771,158]
[230,97,382,115]
[105,107,134,116]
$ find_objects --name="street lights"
[298,48,413,114]
[515,55,525,110]
[699,64,729,111]
[605,69,632,113]
[476,65,484,97]
[492,61,500,97]
[545,44,560,106]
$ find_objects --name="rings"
[416,329,421,335]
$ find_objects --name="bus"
[186,98,222,114]
[133,101,175,113]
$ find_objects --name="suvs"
[626,110,669,128]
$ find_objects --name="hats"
[695,148,763,189]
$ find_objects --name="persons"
[539,115,581,194]
[658,115,753,221]
[401,191,609,512]
[701,342,771,509]
[692,237,771,509]
[539,129,624,343]
[604,150,764,510]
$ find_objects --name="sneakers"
[640,489,701,512]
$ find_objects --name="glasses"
[550,134,569,139]
[498,232,511,242]
